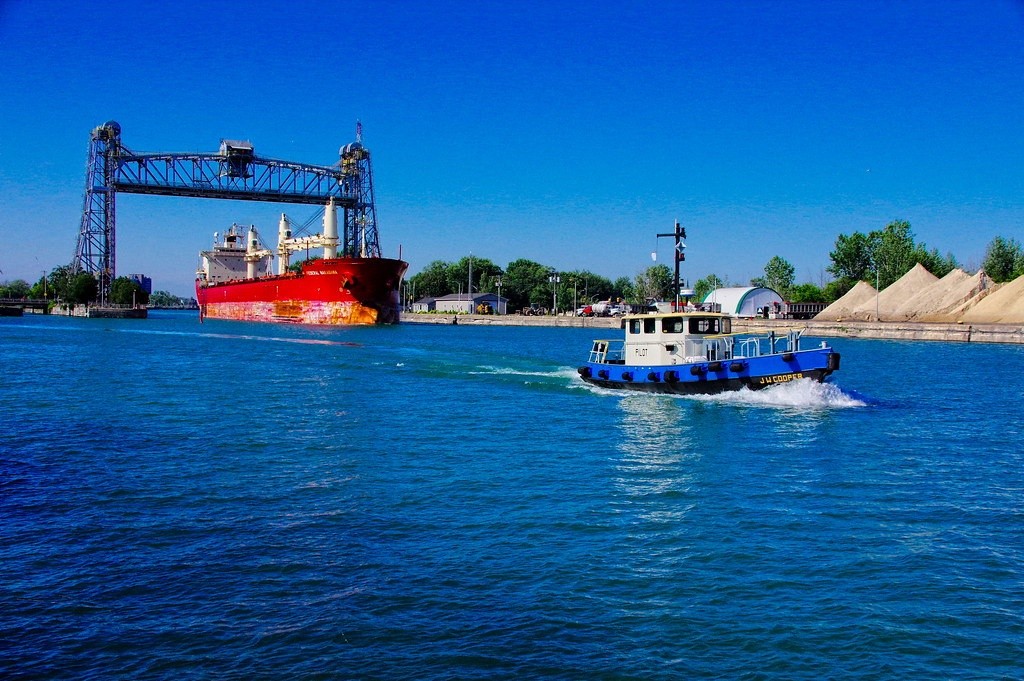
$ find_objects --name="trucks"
[647,301,672,314]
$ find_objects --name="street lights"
[451,279,461,315]
[494,275,503,315]
[548,273,561,316]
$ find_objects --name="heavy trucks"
[609,302,659,316]
[582,301,613,316]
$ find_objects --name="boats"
[578,219,842,397]
[195,195,409,325]
